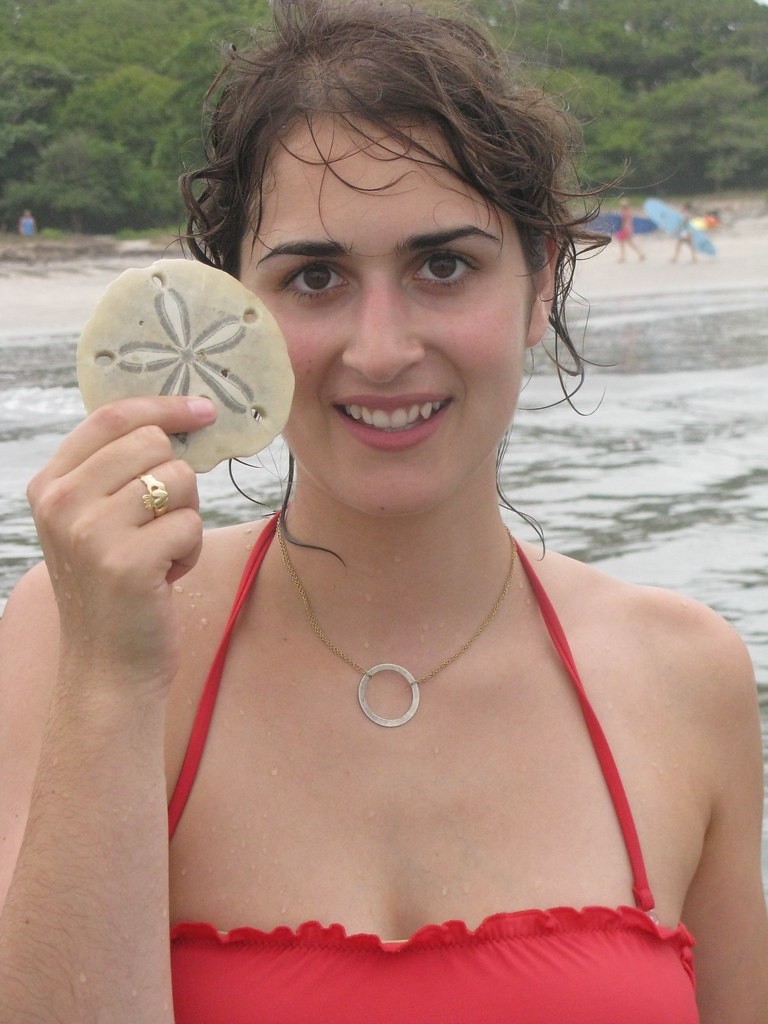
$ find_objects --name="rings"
[139,474,168,516]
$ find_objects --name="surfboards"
[585,213,657,234]
[643,197,716,255]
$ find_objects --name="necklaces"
[276,517,515,727]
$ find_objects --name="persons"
[669,203,698,262]
[0,0,768,1024]
[614,198,645,262]
[18,210,37,235]
